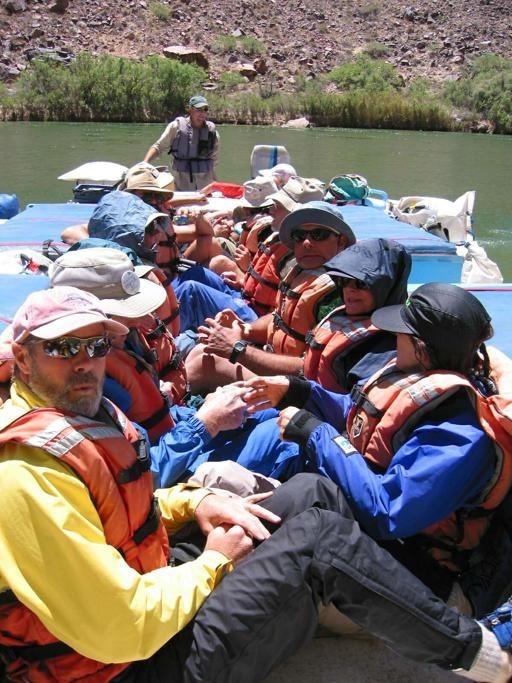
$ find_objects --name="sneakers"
[479,597,512,650]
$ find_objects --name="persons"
[47,247,247,486]
[177,201,358,395]
[183,177,280,280]
[237,281,512,577]
[88,190,251,358]
[204,164,296,237]
[0,286,512,683]
[67,238,209,407]
[170,177,336,332]
[205,238,412,450]
[126,161,214,224]
[145,96,221,191]
[60,175,225,273]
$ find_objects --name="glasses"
[290,227,333,243]
[141,193,168,202]
[192,107,209,113]
[23,335,112,359]
[334,277,368,290]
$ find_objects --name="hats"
[239,176,279,207]
[132,263,155,279]
[12,285,130,340]
[146,211,169,227]
[189,95,210,110]
[49,247,167,318]
[371,281,492,371]
[123,162,174,201]
[279,201,356,250]
[258,162,297,177]
[125,162,174,188]
[265,176,326,213]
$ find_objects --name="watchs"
[228,338,250,364]
